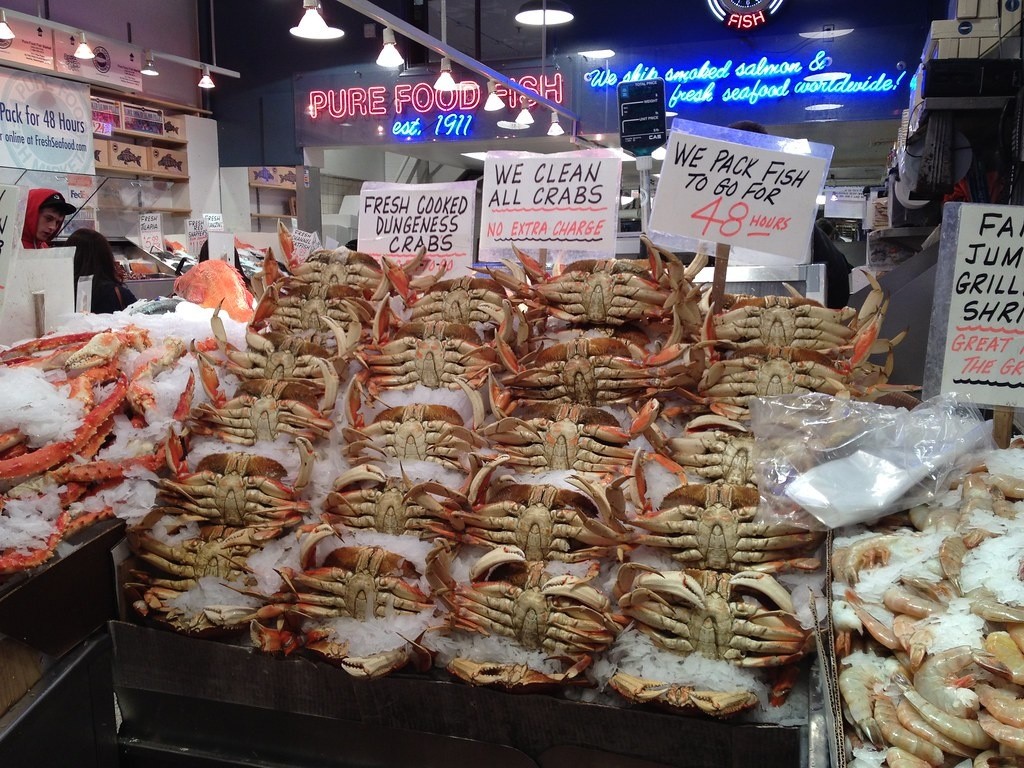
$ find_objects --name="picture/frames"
[90,96,121,129]
[120,102,163,136]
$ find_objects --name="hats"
[39,194,77,215]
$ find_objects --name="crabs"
[0,240,256,573]
[186,214,423,449]
[138,426,315,531]
[121,525,312,631]
[228,234,924,717]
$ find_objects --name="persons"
[65,228,137,313]
[702,122,851,310]
[20,189,77,249]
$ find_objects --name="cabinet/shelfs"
[248,165,298,233]
[0,518,129,766]
[88,86,191,242]
[867,98,1024,276]
[117,561,797,768]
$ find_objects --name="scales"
[615,77,707,264]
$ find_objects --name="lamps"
[0,9,217,89]
[291,0,582,141]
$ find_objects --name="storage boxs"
[93,139,187,175]
[164,116,184,138]
[249,166,295,187]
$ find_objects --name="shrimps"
[827,435,1023,768]
